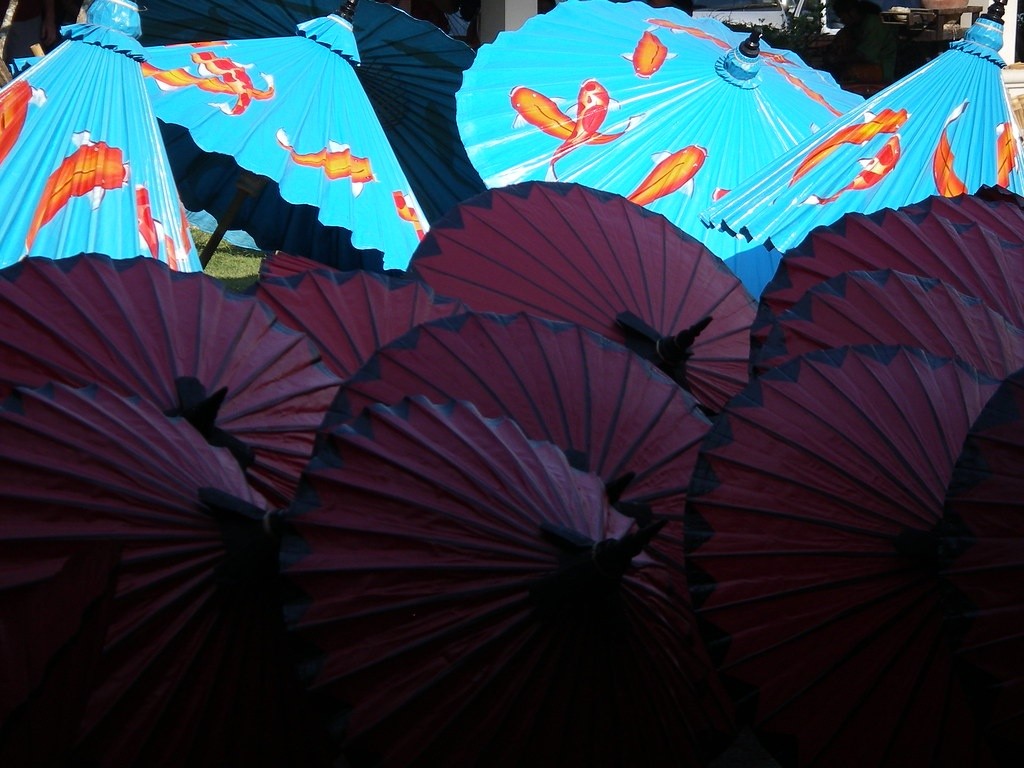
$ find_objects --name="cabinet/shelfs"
[878,5,984,70]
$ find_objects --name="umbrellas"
[750,268,1024,412]
[682,343,1003,768]
[0,383,288,768]
[699,0,1024,257]
[0,0,487,272]
[407,181,757,423]
[244,267,473,381]
[274,311,713,768]
[932,365,1024,768]
[454,0,866,301]
[973,183,1024,212]
[749,193,1024,379]
[9,0,431,272]
[0,252,345,510]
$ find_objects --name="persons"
[0,1,203,275]
[820,0,896,85]
